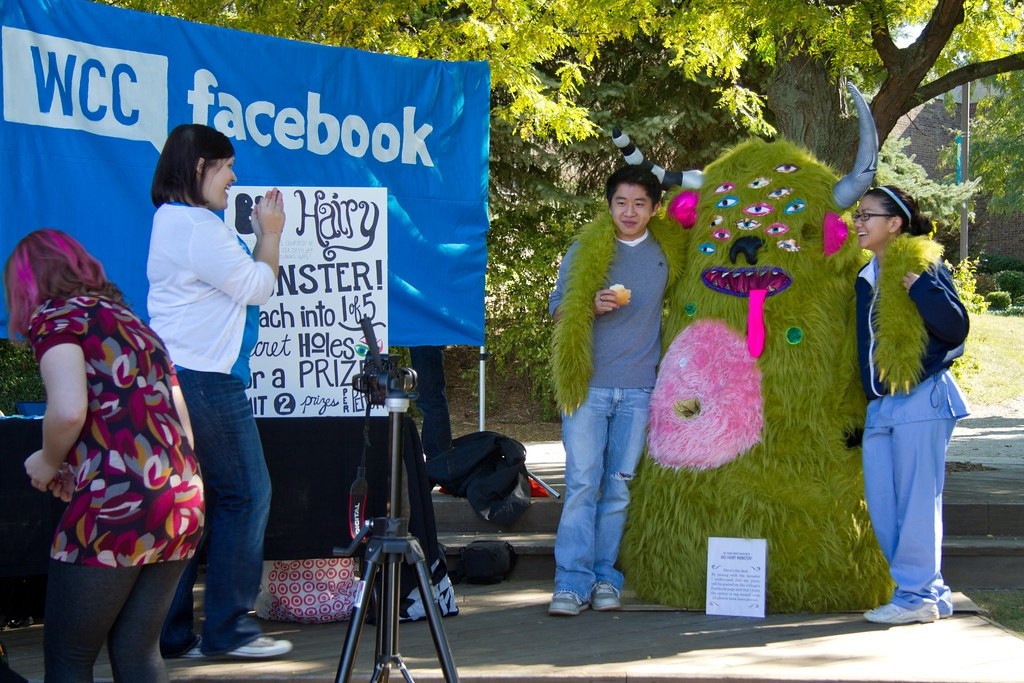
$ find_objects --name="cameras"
[364,354,396,404]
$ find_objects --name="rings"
[600,295,603,300]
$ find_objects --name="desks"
[0,415,459,631]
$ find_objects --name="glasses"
[852,213,893,222]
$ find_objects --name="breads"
[609,283,631,305]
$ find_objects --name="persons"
[147,124,293,658]
[854,185,970,625]
[5,228,205,683]
[409,345,452,486]
[549,165,668,616]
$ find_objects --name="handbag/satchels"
[448,541,518,585]
[253,558,356,624]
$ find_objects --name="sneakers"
[181,634,207,658]
[548,592,589,616]
[863,603,949,623]
[226,636,293,657]
[590,583,621,610]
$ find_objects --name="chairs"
[15,401,47,416]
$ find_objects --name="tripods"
[333,317,460,683]
